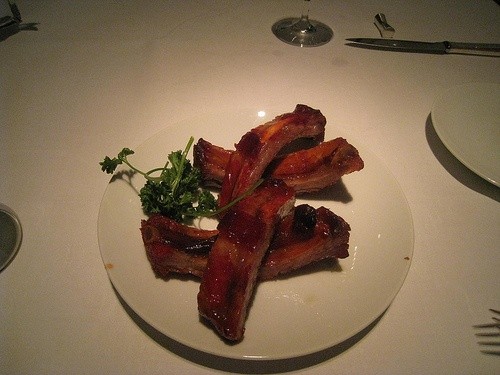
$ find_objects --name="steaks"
[139,104,364,344]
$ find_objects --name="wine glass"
[271,0,334,49]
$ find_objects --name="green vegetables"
[99,136,266,222]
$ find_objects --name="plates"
[430,81,499,189]
[96,112,414,360]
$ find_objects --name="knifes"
[344,38,499,56]
